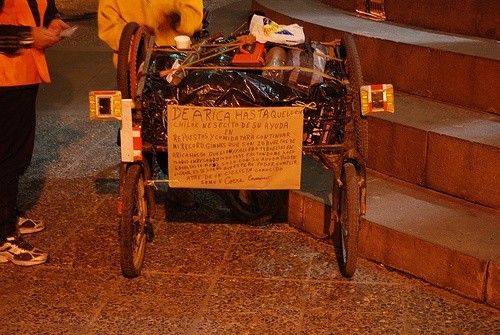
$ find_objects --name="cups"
[174,35,190,48]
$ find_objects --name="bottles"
[262,46,287,83]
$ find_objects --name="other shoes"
[168,189,196,206]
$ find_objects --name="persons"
[97,0,203,207]
[0,0,71,266]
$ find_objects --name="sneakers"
[0,229,49,266]
[16,215,46,234]
